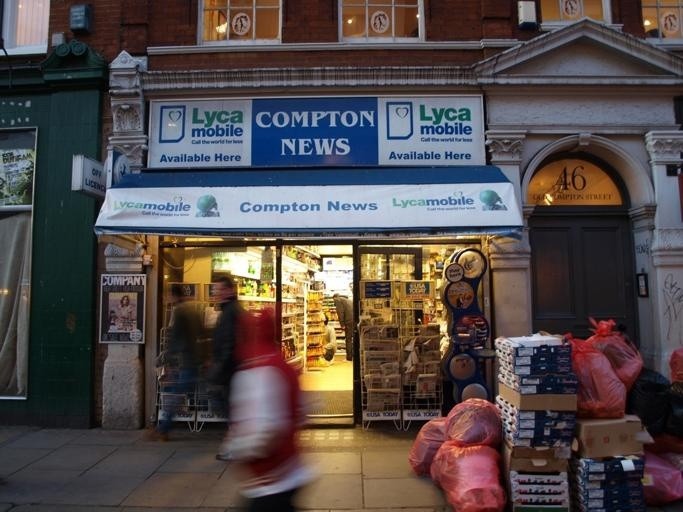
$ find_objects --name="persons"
[331,293,354,364]
[114,294,134,331]
[204,270,250,421]
[217,304,321,512]
[139,279,203,436]
[323,316,338,366]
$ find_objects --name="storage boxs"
[495,334,656,511]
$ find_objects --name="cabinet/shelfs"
[235,244,354,375]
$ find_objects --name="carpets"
[300,390,353,414]
